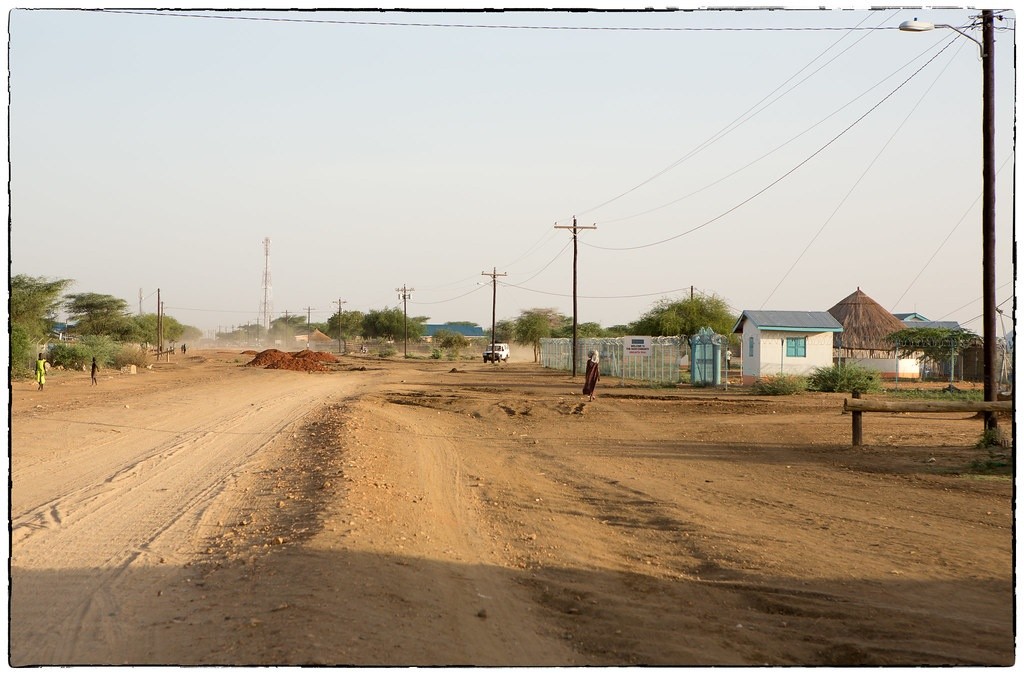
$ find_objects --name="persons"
[583,349,600,401]
[91,357,100,385]
[181,343,186,353]
[498,346,501,351]
[306,342,310,349]
[725,348,732,370]
[35,353,47,391]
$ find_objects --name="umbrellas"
[827,287,915,351]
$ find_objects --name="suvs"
[482,341,510,363]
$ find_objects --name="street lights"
[477,283,496,363]
[900,21,995,439]
